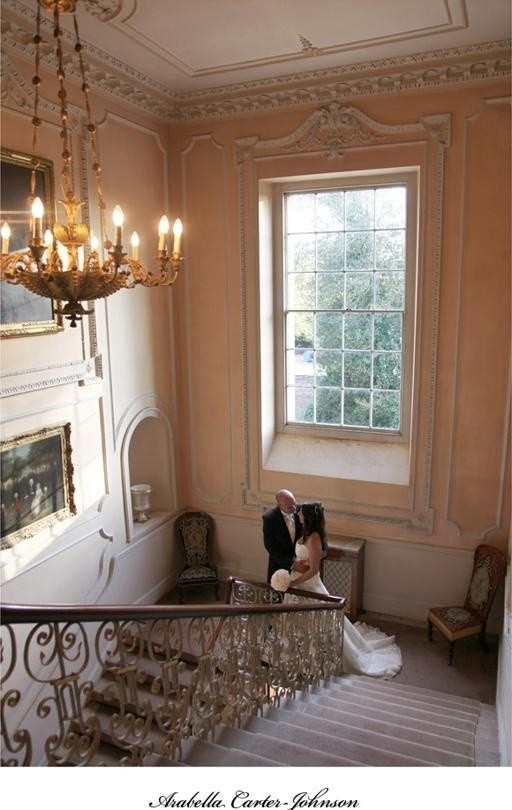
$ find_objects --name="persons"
[29,482,46,518]
[44,485,49,507]
[0,503,6,530]
[255,501,330,679]
[11,490,22,522]
[24,477,35,510]
[261,488,328,585]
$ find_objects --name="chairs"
[174,511,221,604]
[424,543,507,666]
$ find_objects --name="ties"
[284,514,296,544]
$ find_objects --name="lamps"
[0,1,187,329]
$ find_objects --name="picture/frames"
[0,146,66,340]
[0,420,79,552]
